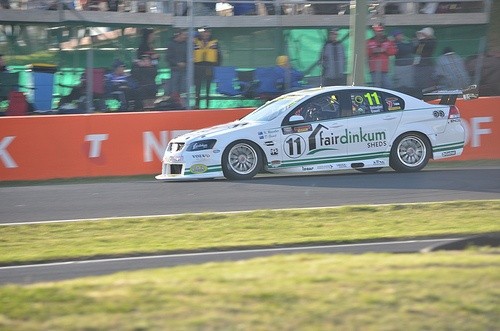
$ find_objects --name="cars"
[155,85,465,180]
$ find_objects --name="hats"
[418,27,434,37]
[373,25,385,31]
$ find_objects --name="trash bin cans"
[32,61,58,111]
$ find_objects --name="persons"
[294,98,337,123]
[319,28,345,86]
[137,28,161,94]
[435,47,473,98]
[192,28,222,109]
[413,28,436,91]
[104,58,143,112]
[393,28,418,89]
[274,54,308,88]
[166,28,189,97]
[0,53,10,72]
[365,24,398,89]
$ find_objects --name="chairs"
[5,66,313,116]
